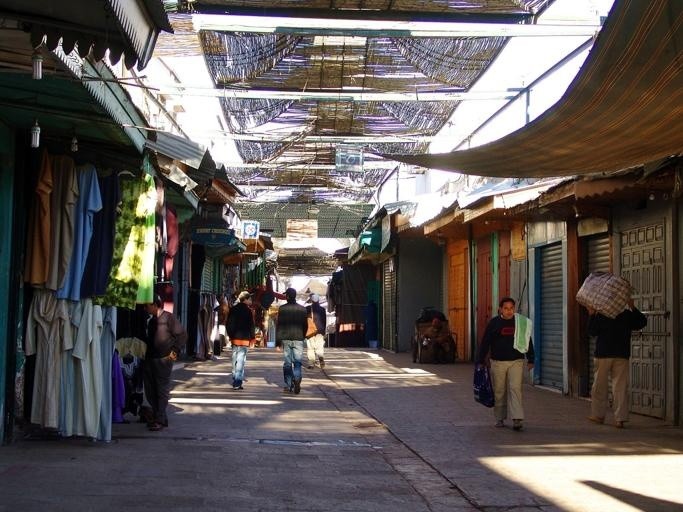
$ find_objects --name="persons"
[225,290,255,391]
[475,295,535,431]
[358,299,376,350]
[141,294,188,432]
[274,287,308,395]
[583,298,647,428]
[304,292,328,370]
[422,318,451,364]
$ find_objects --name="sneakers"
[494,420,505,428]
[234,379,242,389]
[284,387,293,393]
[586,412,605,423]
[318,357,326,368]
[616,419,624,428]
[307,364,314,369]
[294,382,302,395]
[512,418,524,429]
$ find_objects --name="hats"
[283,289,296,296]
[311,294,318,303]
[239,292,256,300]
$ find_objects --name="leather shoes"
[148,419,168,432]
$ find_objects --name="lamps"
[29,117,40,149]
[30,47,45,81]
[70,136,79,153]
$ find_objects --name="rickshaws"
[411,306,458,365]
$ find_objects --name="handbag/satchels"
[576,270,631,318]
[473,362,496,409]
[305,317,318,340]
